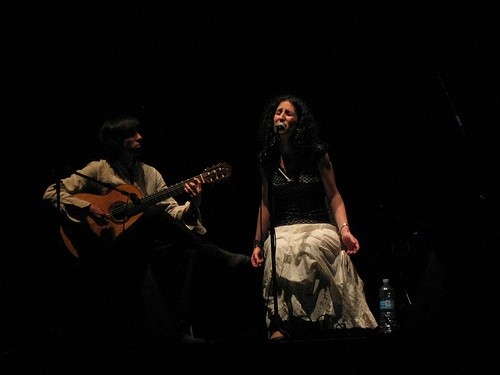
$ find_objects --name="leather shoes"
[212,248,249,268]
[178,332,206,345]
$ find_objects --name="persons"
[250,95,379,341]
[42,115,250,346]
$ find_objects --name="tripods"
[210,129,295,345]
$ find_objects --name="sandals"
[270,330,287,341]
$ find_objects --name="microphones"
[130,195,141,206]
[276,123,286,131]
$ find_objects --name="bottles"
[379,278,396,330]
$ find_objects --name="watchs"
[338,222,350,233]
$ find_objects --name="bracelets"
[252,240,263,248]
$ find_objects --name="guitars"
[58,161,235,262]
